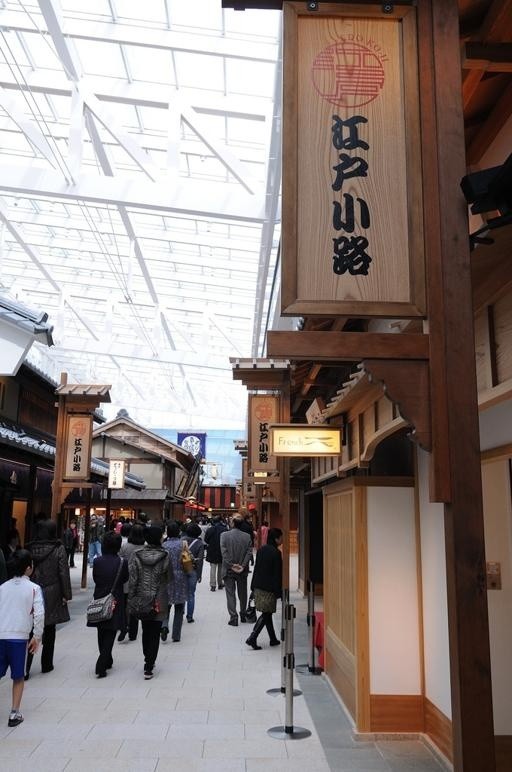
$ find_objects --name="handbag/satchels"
[245,598,258,623]
[85,593,118,624]
[180,539,194,574]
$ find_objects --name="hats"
[92,516,96,520]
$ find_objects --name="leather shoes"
[161,626,170,641]
[218,585,225,589]
[210,585,217,591]
[228,620,238,626]
[241,616,246,623]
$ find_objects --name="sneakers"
[188,620,194,623]
[97,672,107,678]
[118,629,128,641]
[143,671,154,679]
[7,710,25,728]
[39,665,54,674]
[23,670,31,681]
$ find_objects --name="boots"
[264,618,281,646]
[245,615,265,650]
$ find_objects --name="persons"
[220,515,252,626]
[110,514,204,679]
[245,527,282,650]
[93,531,129,677]
[61,514,107,568]
[1,515,72,726]
[185,514,270,592]
